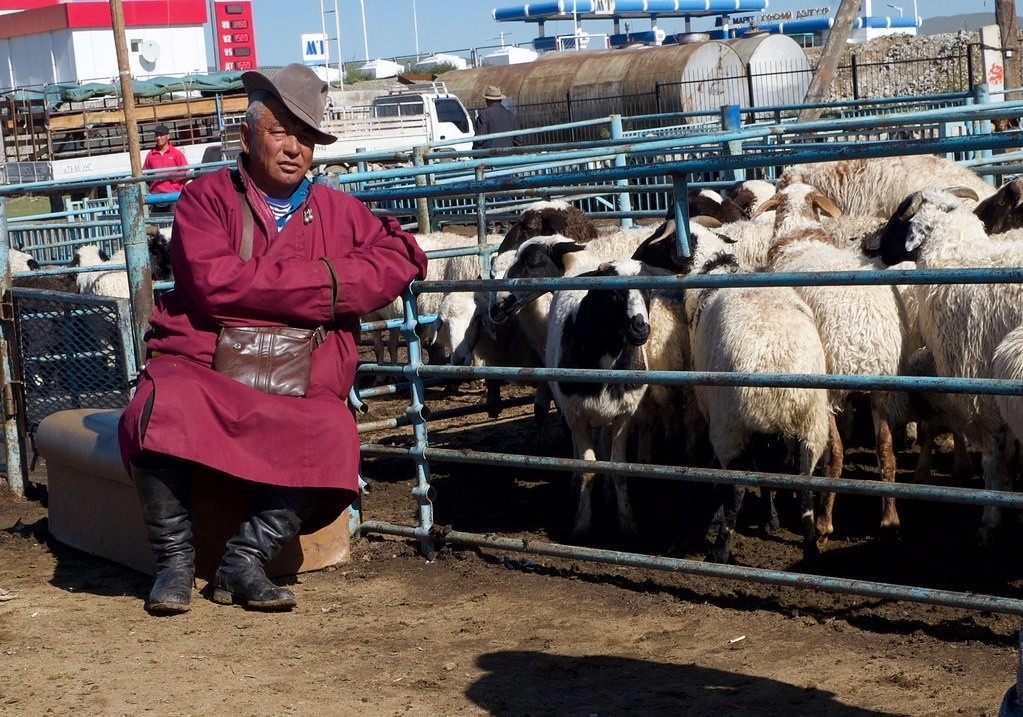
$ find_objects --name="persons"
[471,86,525,162]
[140,125,188,228]
[117,63,432,614]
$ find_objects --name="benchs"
[36,407,354,590]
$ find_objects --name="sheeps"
[9,154,1023,580]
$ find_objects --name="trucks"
[219,81,476,174]
[0,70,250,186]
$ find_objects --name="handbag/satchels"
[215,321,315,399]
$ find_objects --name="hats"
[482,85,507,100]
[241,60,339,144]
[152,126,169,134]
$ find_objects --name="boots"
[131,450,198,613]
[213,497,304,611]
